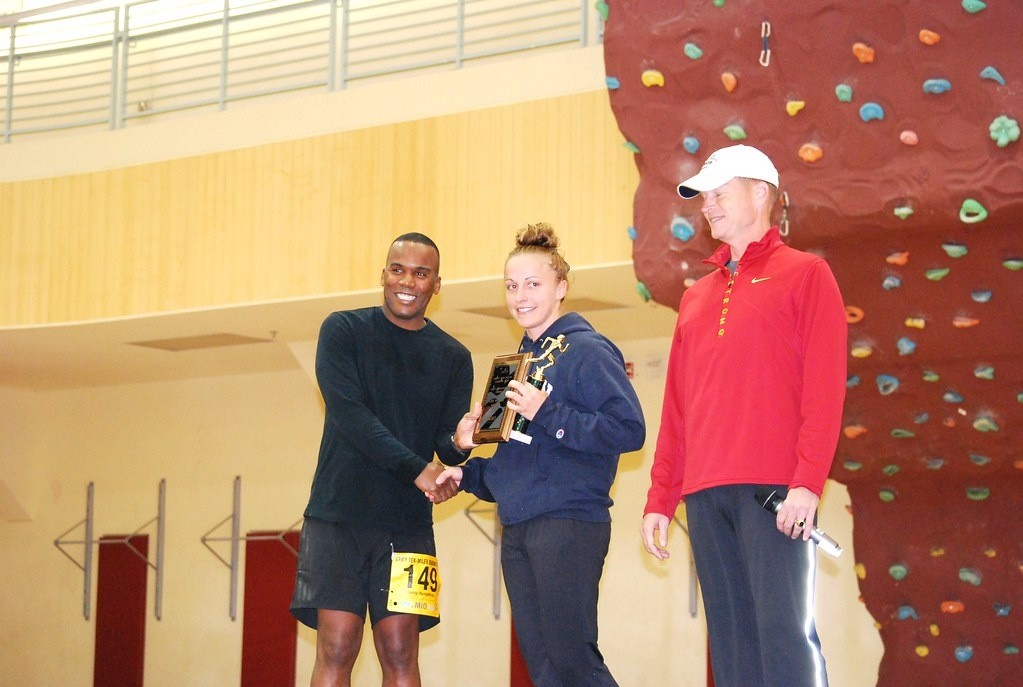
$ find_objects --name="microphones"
[753,485,843,557]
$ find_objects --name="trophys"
[512,333,569,434]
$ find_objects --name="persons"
[639,145,847,687]
[289,233,481,687]
[426,222,647,687]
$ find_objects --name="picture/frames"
[472,352,534,443]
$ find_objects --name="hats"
[677,144,779,200]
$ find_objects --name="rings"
[795,519,804,527]
